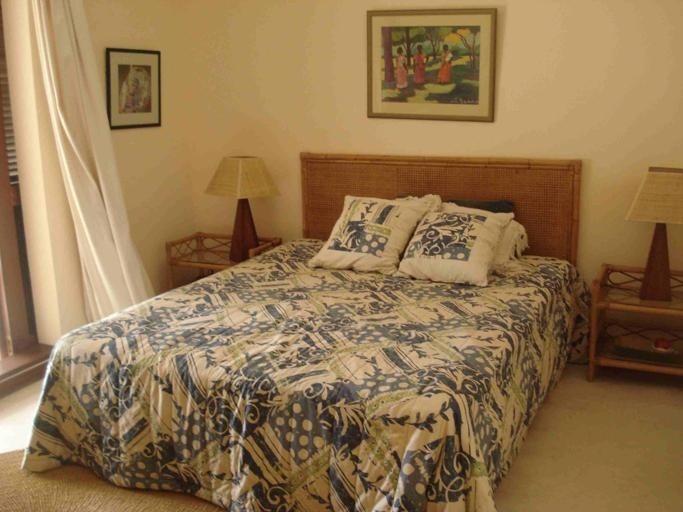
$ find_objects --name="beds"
[53,151,581,512]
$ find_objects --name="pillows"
[308,194,527,287]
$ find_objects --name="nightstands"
[587,263,682,380]
[164,231,281,293]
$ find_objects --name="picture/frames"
[104,47,161,130]
[366,8,497,122]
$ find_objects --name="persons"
[396,44,454,91]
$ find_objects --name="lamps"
[205,154,272,262]
[622,167,682,302]
